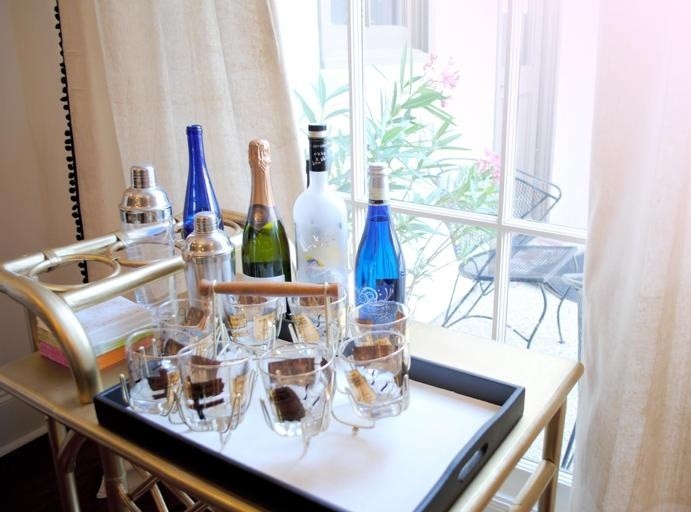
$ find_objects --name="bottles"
[241,137,291,313]
[180,210,237,311]
[355,163,407,318]
[180,124,225,235]
[291,122,351,302]
[122,162,178,319]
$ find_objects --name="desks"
[1,314,585,512]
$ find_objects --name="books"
[36,295,152,371]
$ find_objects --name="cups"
[159,298,217,355]
[259,342,333,435]
[349,299,413,347]
[175,339,254,433]
[335,333,414,419]
[283,289,351,349]
[223,291,284,357]
[123,327,196,415]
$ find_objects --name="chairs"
[428,157,579,350]
